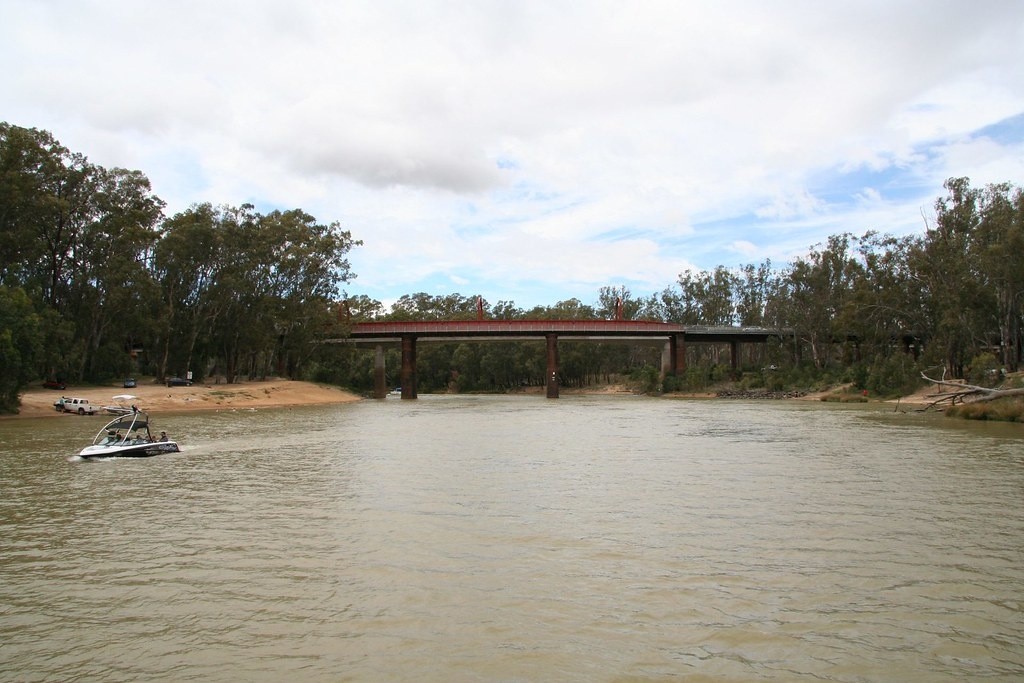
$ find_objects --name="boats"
[80,408,179,459]
[106,395,145,414]
[391,388,402,395]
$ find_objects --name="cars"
[43,381,67,390]
[124,378,136,388]
[165,378,193,387]
[761,365,778,372]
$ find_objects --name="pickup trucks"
[54,397,102,416]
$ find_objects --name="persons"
[60,396,65,414]
[109,429,168,442]
